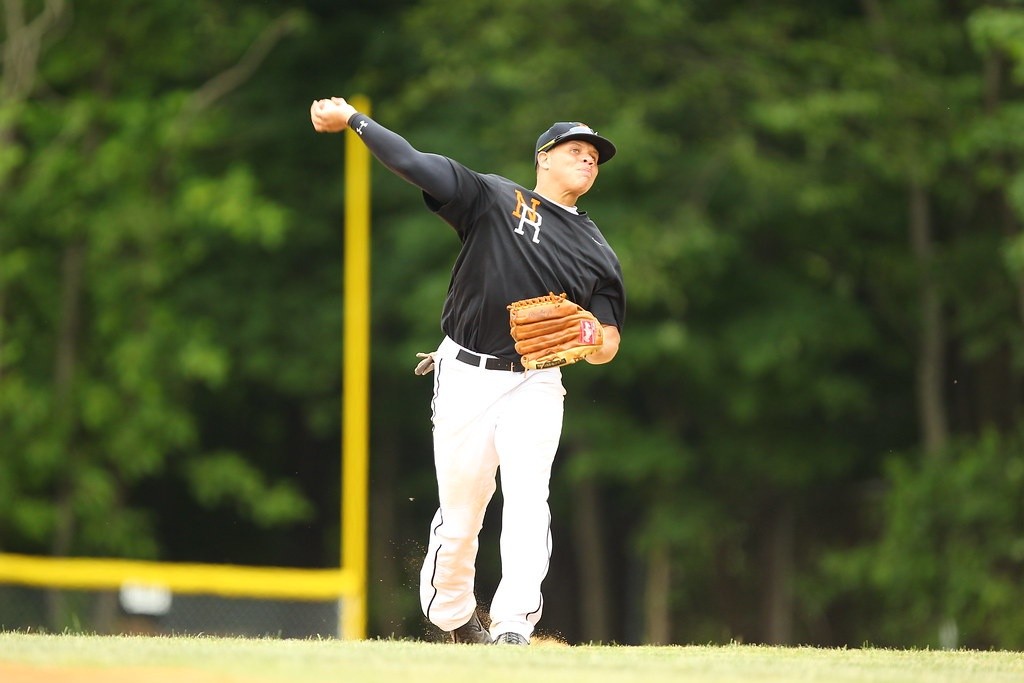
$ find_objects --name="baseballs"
[318,98,337,111]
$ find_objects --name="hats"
[535,121,616,172]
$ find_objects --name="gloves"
[414,352,437,376]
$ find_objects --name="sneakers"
[495,633,529,646]
[450,609,493,646]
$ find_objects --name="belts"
[455,350,529,373]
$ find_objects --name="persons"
[308,97,625,647]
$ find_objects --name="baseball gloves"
[507,291,606,379]
